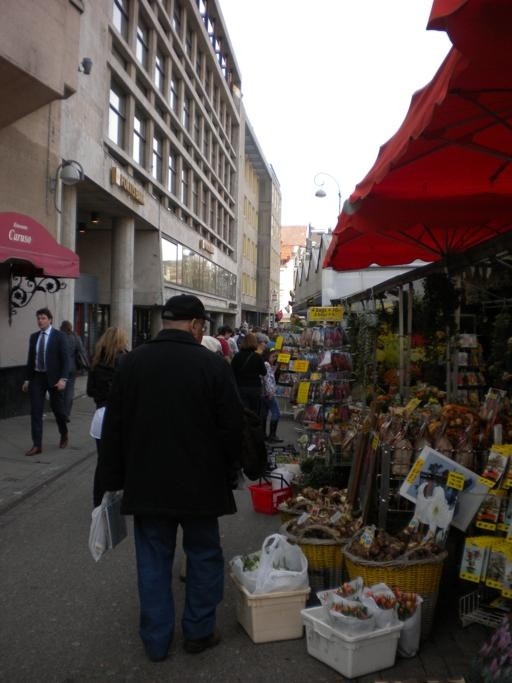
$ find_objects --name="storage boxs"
[228,547,404,680]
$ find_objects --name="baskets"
[279,519,350,608]
[341,546,447,639]
[278,501,304,526]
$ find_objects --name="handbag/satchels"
[76,345,90,370]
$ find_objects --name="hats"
[257,336,270,343]
[162,294,215,322]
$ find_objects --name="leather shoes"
[26,446,41,456]
[185,634,220,653]
[59,433,67,448]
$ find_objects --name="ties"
[38,332,44,371]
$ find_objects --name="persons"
[61,320,89,423]
[200,324,294,444]
[89,292,246,661]
[22,308,71,455]
[87,325,130,504]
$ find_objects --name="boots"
[268,420,284,443]
